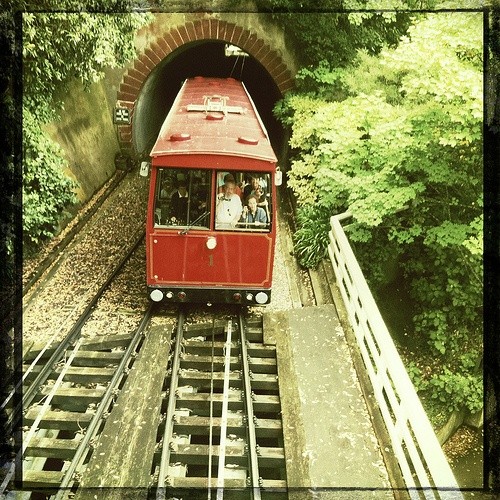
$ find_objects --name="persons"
[216,181,243,229]
[218,173,269,206]
[240,195,266,229]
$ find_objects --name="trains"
[141,73,282,305]
